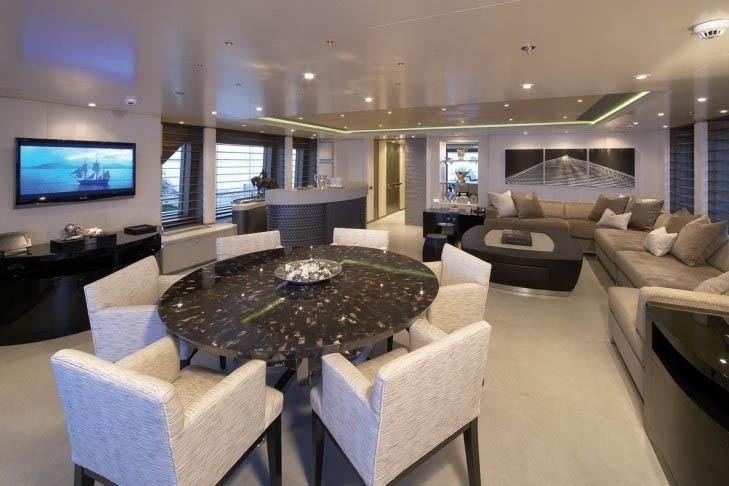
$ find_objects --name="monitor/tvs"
[13,138,136,209]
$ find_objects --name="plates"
[273,258,343,284]
[272,280,340,302]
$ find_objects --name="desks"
[422,207,486,241]
[156,242,439,447]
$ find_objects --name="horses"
[251,176,278,199]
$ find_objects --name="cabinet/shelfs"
[0,229,161,347]
[640,300,729,485]
[441,144,478,197]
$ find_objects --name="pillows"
[511,190,545,218]
[643,226,678,257]
[624,198,665,230]
[653,213,702,234]
[671,214,729,268]
[486,189,518,218]
[597,208,632,230]
[694,271,728,296]
[588,193,631,220]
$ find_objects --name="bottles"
[456,191,469,203]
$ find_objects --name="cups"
[327,177,344,187]
[456,149,465,160]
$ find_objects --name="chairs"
[329,227,392,253]
[387,243,493,352]
[49,333,286,486]
[308,318,491,486]
[215,229,282,262]
[84,254,228,373]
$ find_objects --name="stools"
[436,223,457,246]
[422,233,448,262]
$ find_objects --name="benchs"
[155,222,239,276]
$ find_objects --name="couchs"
[483,196,729,403]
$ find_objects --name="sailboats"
[71,152,111,186]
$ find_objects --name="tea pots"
[313,174,327,189]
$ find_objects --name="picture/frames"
[506,149,635,190]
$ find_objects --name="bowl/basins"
[455,170,468,179]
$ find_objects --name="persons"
[258,169,268,186]
